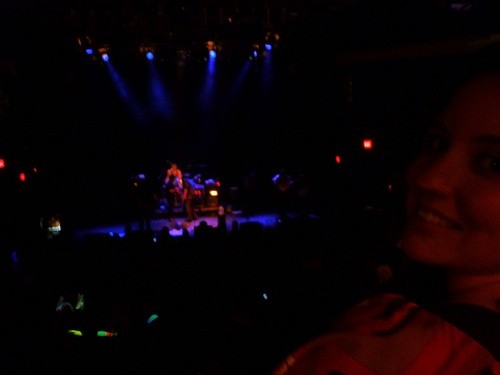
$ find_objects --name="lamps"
[176,45,191,65]
[39,214,63,239]
[245,31,281,61]
[136,38,156,62]
[202,38,219,60]
[75,36,111,64]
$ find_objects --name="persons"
[283,76,500,375]
[165,164,183,191]
[182,173,198,222]
